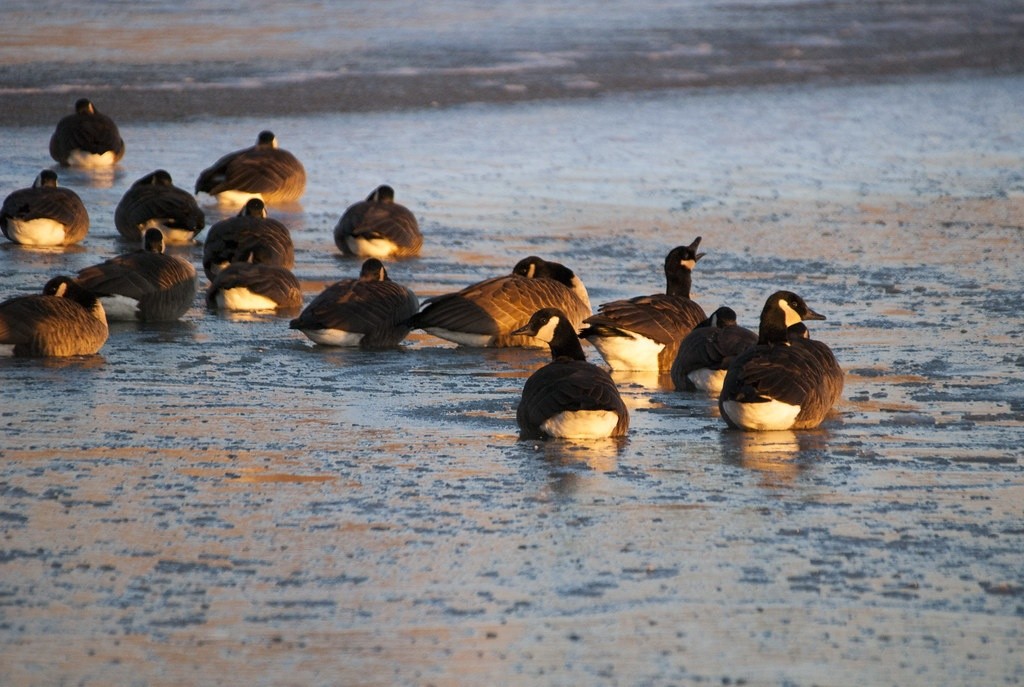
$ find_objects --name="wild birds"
[509,307,630,439]
[194,130,306,204]
[718,291,845,431]
[113,169,205,241]
[48,99,126,165]
[74,228,199,321]
[286,258,420,349]
[334,185,423,258]
[206,251,303,311]
[1,168,90,246]
[394,256,593,350]
[0,276,109,356]
[576,237,708,372]
[203,198,294,284]
[671,307,760,394]
